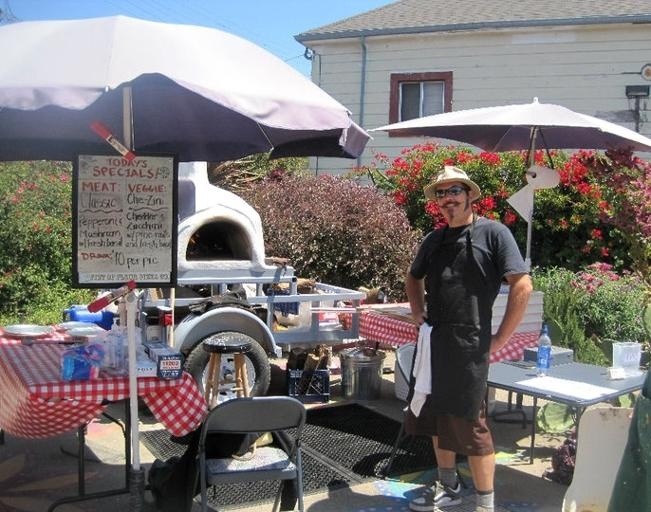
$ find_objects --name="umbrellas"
[0,15,372,512]
[366,97,651,272]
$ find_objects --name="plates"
[3,325,53,336]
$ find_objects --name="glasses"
[435,185,467,198]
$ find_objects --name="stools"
[203,335,252,411]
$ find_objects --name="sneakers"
[409,479,463,512]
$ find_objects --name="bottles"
[537,328,552,376]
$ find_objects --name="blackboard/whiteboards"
[72,152,178,288]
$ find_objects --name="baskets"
[286,363,330,403]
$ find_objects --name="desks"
[332,302,540,429]
[0,323,210,512]
[487,361,649,463]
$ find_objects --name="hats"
[423,165,480,200]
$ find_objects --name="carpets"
[137,403,468,512]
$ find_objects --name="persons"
[405,165,533,511]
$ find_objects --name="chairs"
[382,343,467,490]
[187,396,304,512]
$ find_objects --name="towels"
[410,322,432,418]
